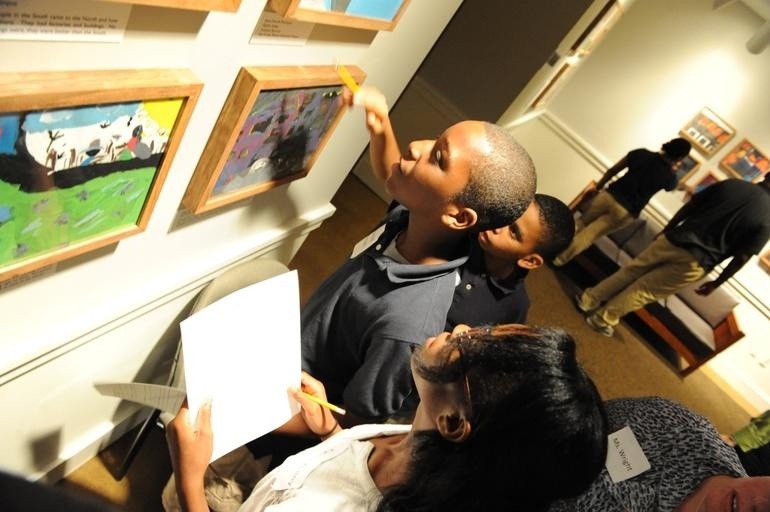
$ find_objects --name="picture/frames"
[179,64,366,216]
[718,138,770,182]
[674,155,702,190]
[108,1,241,15]
[0,65,207,278]
[570,0,625,55]
[678,106,735,159]
[760,250,769,271]
[269,0,410,32]
[532,65,576,109]
[689,171,719,193]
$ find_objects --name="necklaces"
[696,475,730,511]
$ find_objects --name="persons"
[265,87,537,471]
[718,410,770,477]
[163,320,609,512]
[574,171,770,337]
[549,396,770,511]
[552,137,692,268]
[444,193,576,333]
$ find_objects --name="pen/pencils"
[336,62,360,93]
[302,392,347,416]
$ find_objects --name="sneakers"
[586,314,615,338]
[575,290,588,313]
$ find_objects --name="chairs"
[115,257,291,483]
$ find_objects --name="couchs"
[550,182,746,379]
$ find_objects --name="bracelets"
[316,418,339,437]
